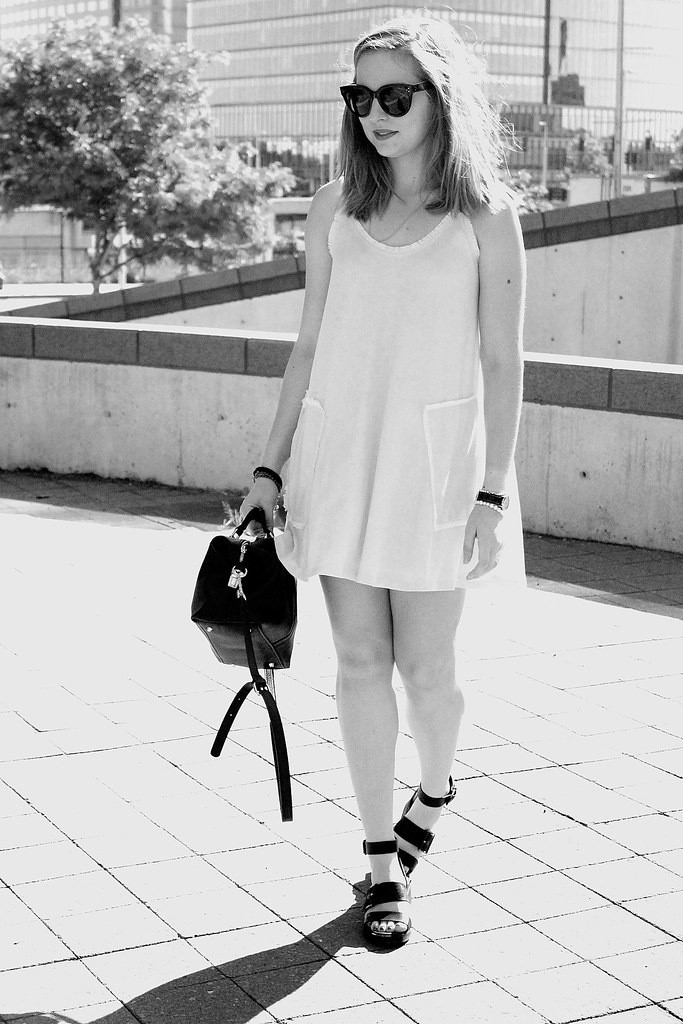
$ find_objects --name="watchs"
[477,491,510,510]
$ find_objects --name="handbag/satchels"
[190,506,298,824]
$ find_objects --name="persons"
[240,16,526,949]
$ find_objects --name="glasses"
[339,83,434,119]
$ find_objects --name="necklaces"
[369,192,434,242]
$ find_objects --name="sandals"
[362,774,457,955]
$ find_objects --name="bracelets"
[474,500,504,516]
[253,467,282,494]
